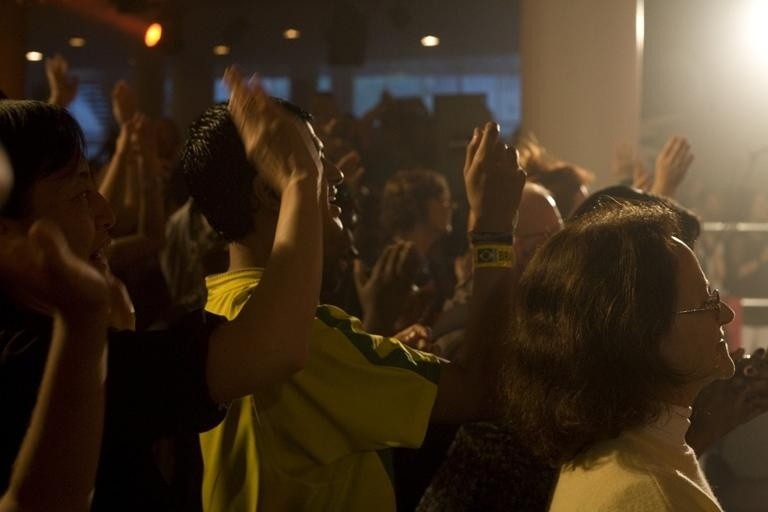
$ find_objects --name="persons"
[0,48,768,511]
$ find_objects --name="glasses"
[676,287,723,321]
